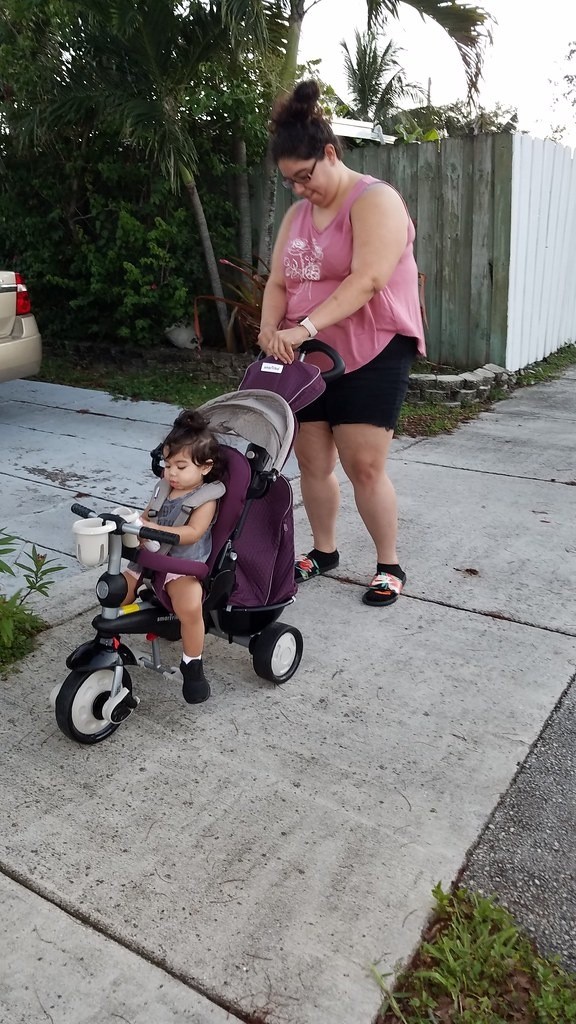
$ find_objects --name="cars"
[0,271,44,385]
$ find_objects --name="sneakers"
[179,656,211,704]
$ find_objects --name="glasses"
[281,147,324,190]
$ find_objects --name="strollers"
[49,339,346,744]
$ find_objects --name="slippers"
[294,551,339,584]
[362,570,407,607]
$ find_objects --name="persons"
[258,76,426,606]
[120,409,229,705]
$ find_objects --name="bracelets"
[297,316,318,338]
